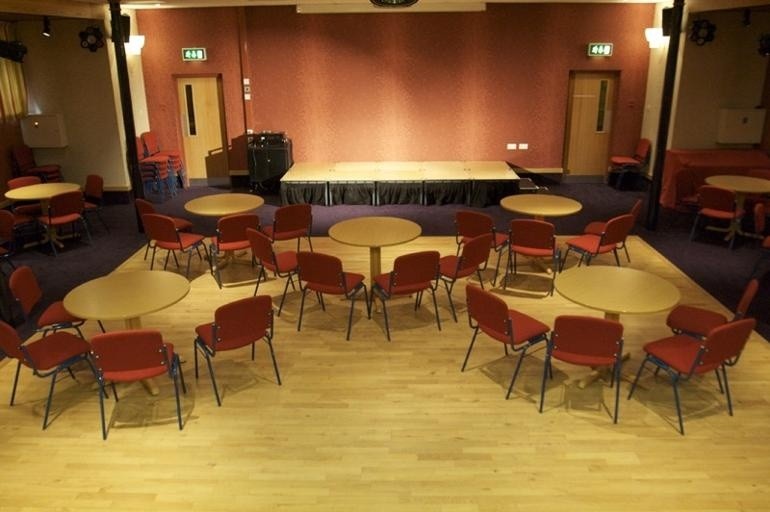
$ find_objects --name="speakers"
[249,143,290,187]
[111,15,131,42]
[662,8,675,37]
